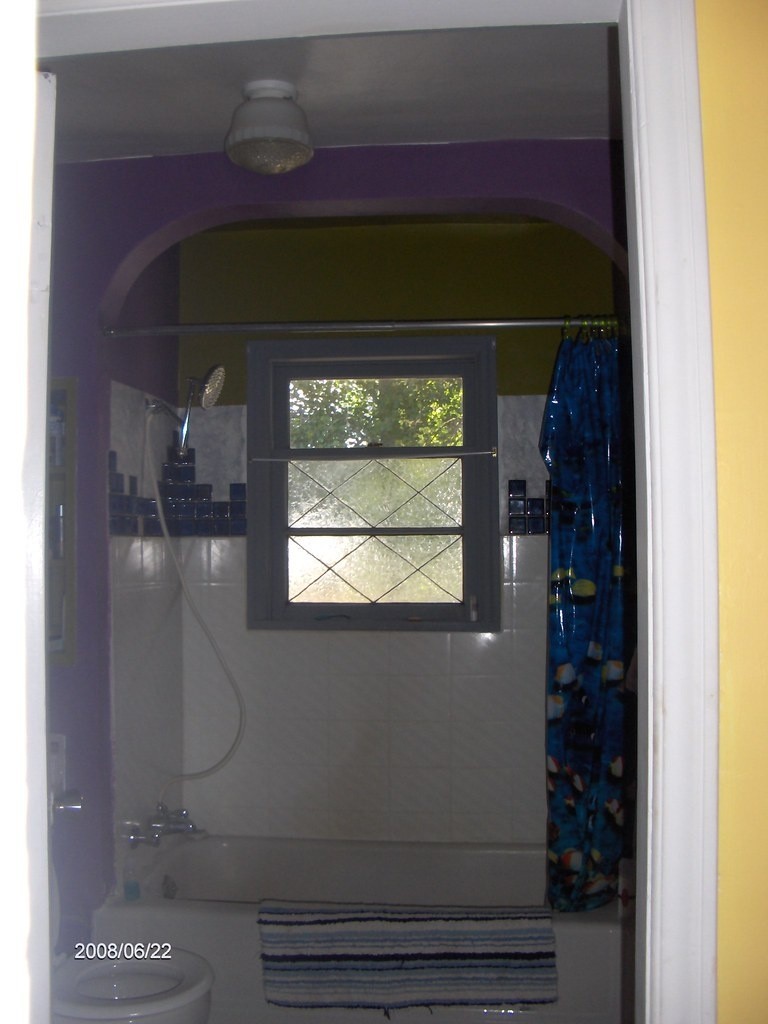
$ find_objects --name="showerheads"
[196,363,226,411]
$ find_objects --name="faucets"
[149,815,195,837]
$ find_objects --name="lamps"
[225,79,315,174]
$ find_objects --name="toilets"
[49,852,214,1024]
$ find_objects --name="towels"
[257,896,557,1011]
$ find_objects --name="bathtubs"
[90,828,623,1024]
